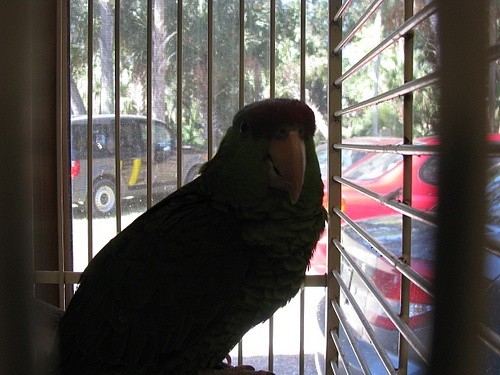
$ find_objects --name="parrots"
[49,98,328,375]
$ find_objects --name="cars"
[70,114,208,215]
[311,133,500,375]
